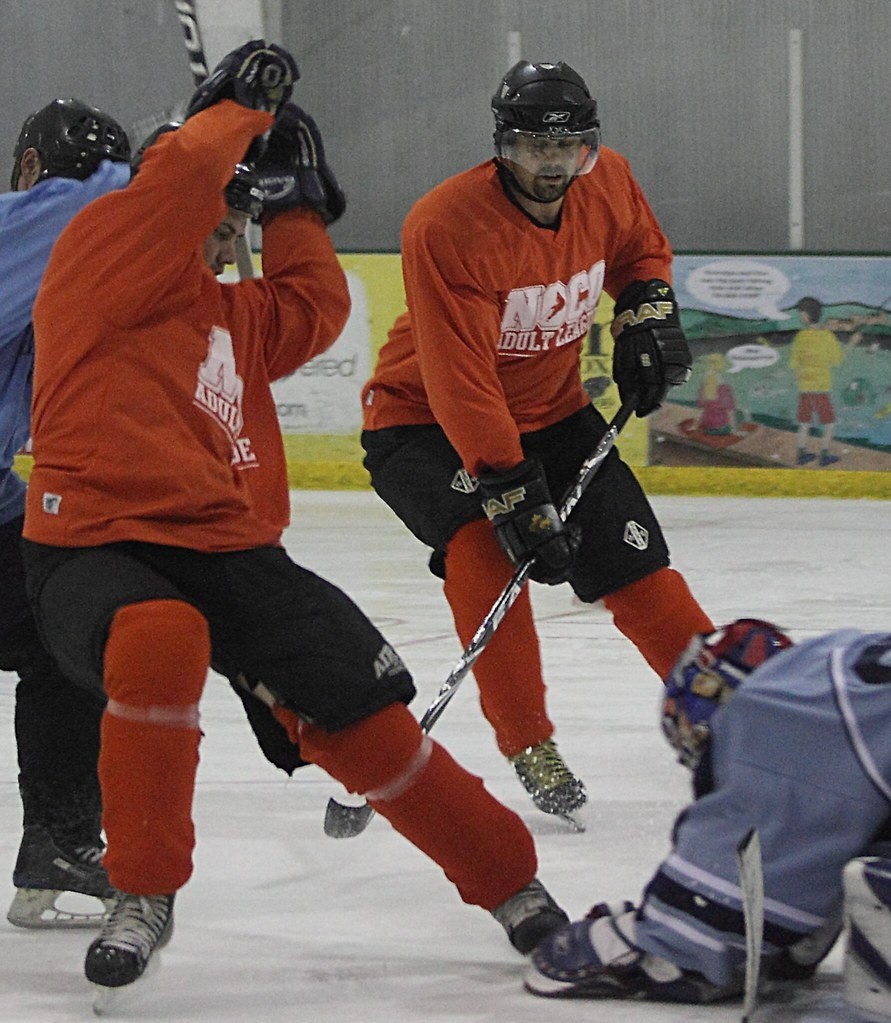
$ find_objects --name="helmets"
[661,618,794,801]
[10,97,132,192]
[130,122,264,220]
[490,60,601,155]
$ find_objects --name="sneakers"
[4,813,123,929]
[488,876,571,956]
[508,738,588,832]
[84,887,175,1015]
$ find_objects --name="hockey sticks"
[323,391,640,839]
[175,1,254,280]
[736,825,766,1023]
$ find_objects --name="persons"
[523,619,891,1023]
[361,63,714,815]
[0,41,572,987]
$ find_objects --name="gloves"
[472,457,581,586]
[250,102,346,225]
[611,279,695,417]
[184,39,300,117]
